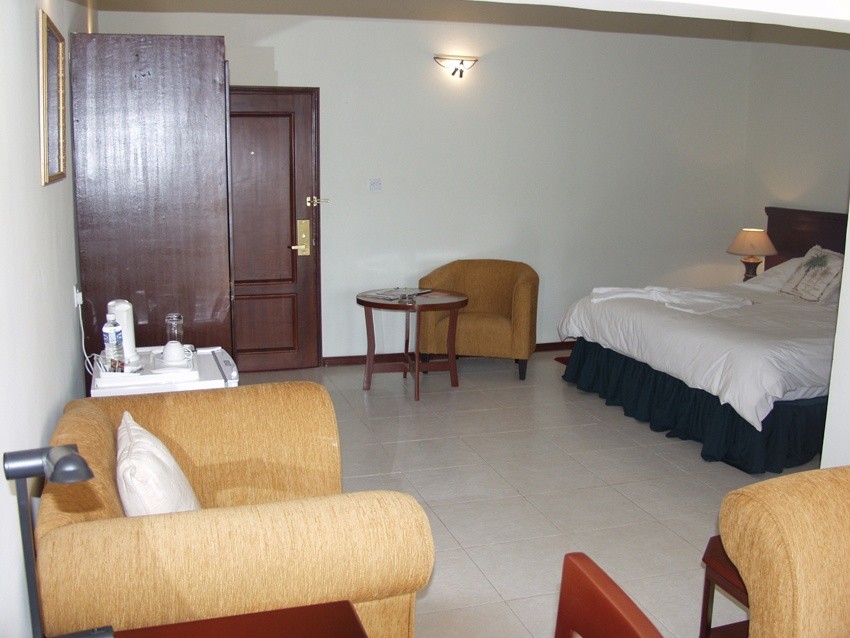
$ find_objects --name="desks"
[113,600,368,638]
[356,288,468,401]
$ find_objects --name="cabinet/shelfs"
[69,32,237,395]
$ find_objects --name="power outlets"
[73,283,81,308]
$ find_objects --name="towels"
[592,284,754,315]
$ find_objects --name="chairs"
[417,259,539,380]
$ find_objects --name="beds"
[555,206,848,473]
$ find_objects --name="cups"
[165,312,184,344]
[163,341,193,364]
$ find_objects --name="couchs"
[716,466,850,638]
[38,381,435,637]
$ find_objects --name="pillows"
[116,410,201,517]
[778,244,844,303]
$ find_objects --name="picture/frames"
[38,9,67,186]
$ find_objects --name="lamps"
[433,57,479,78]
[3,442,114,638]
[725,227,779,282]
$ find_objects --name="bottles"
[102,313,125,364]
